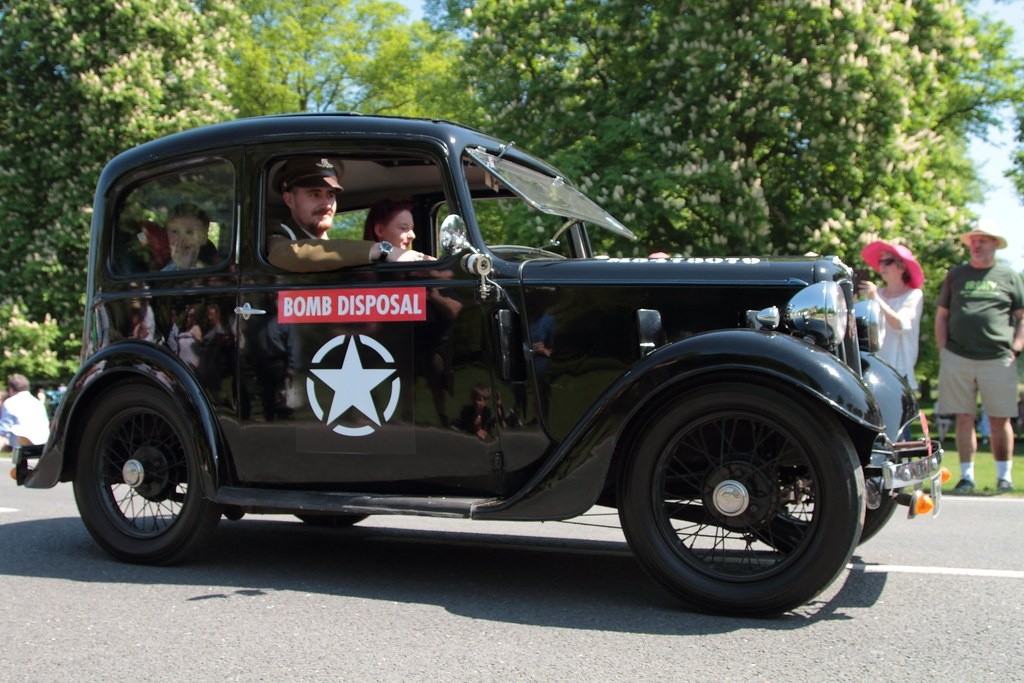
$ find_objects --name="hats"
[274,154,343,193]
[959,224,1007,250]
[863,240,925,289]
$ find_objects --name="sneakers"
[997,479,1013,491]
[953,479,973,492]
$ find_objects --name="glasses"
[878,259,898,266]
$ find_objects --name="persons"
[1,371,69,454]
[154,201,213,272]
[934,222,1024,496]
[264,154,438,274]
[853,238,924,441]
[360,197,415,251]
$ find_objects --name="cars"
[11,110,954,620]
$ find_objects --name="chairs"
[137,219,219,273]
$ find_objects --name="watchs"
[1010,347,1021,359]
[375,240,393,262]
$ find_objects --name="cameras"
[851,269,870,294]
